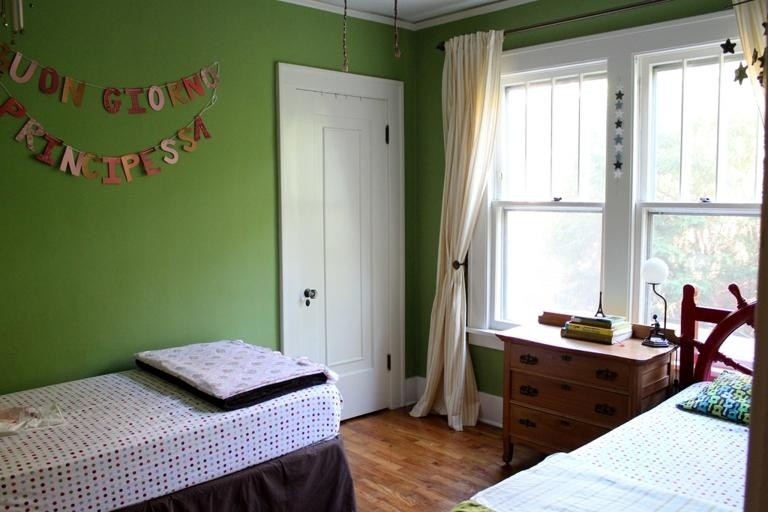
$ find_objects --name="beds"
[450,282,757,511]
[1,366,348,511]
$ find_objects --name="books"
[561,313,633,345]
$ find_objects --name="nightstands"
[496,321,678,466]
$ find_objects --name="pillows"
[676,369,755,425]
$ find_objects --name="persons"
[645,314,665,340]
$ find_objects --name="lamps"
[640,257,669,348]
